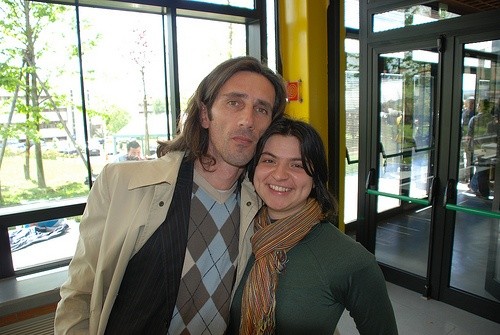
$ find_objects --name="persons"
[222,117,399,335]
[461,95,498,200]
[55,56,287,335]
[127,141,142,157]
